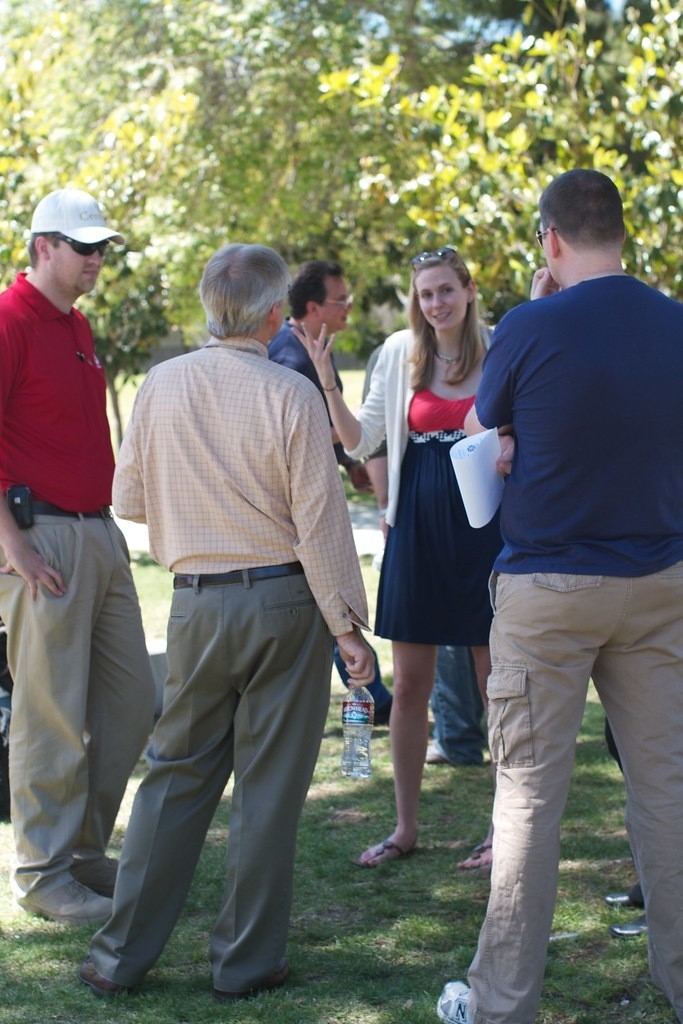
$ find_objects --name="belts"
[27,501,116,519]
[173,559,305,590]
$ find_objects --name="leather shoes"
[611,914,646,939]
[214,957,290,1004]
[77,961,123,999]
[604,891,633,909]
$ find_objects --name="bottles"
[339,686,374,780]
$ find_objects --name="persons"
[0,187,157,925]
[437,169,683,1024]
[291,247,515,868]
[78,242,375,1001]
[266,260,486,768]
[605,715,647,940]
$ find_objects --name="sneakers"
[88,856,126,895]
[16,879,113,923]
[437,981,472,1024]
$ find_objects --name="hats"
[30,188,127,248]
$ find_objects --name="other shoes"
[424,741,448,763]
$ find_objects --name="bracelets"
[321,385,337,393]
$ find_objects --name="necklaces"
[435,351,461,364]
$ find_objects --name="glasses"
[47,233,108,255]
[315,297,352,312]
[411,248,469,275]
[534,227,559,249]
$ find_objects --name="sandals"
[352,836,418,865]
[458,844,493,869]
